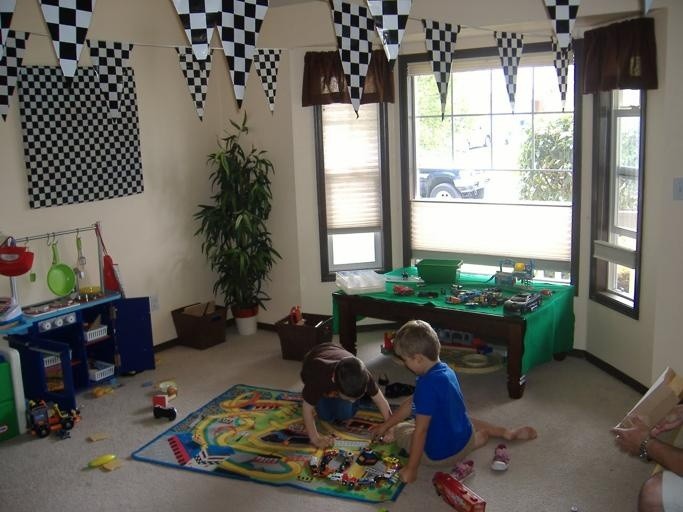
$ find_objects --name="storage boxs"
[169,300,229,350]
[272,310,333,362]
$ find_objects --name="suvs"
[339,139,491,199]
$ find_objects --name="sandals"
[383,382,417,400]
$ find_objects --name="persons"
[299,341,395,450]
[369,319,537,484]
[609,404,682,512]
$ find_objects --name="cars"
[464,103,522,150]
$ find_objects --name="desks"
[324,261,577,400]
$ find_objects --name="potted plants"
[185,107,288,340]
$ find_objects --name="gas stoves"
[23,296,76,335]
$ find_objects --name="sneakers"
[490,442,512,473]
[449,460,476,484]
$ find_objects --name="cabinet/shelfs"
[0,345,31,445]
[0,288,159,426]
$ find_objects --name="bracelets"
[638,434,651,464]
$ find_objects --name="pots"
[47,242,76,298]
